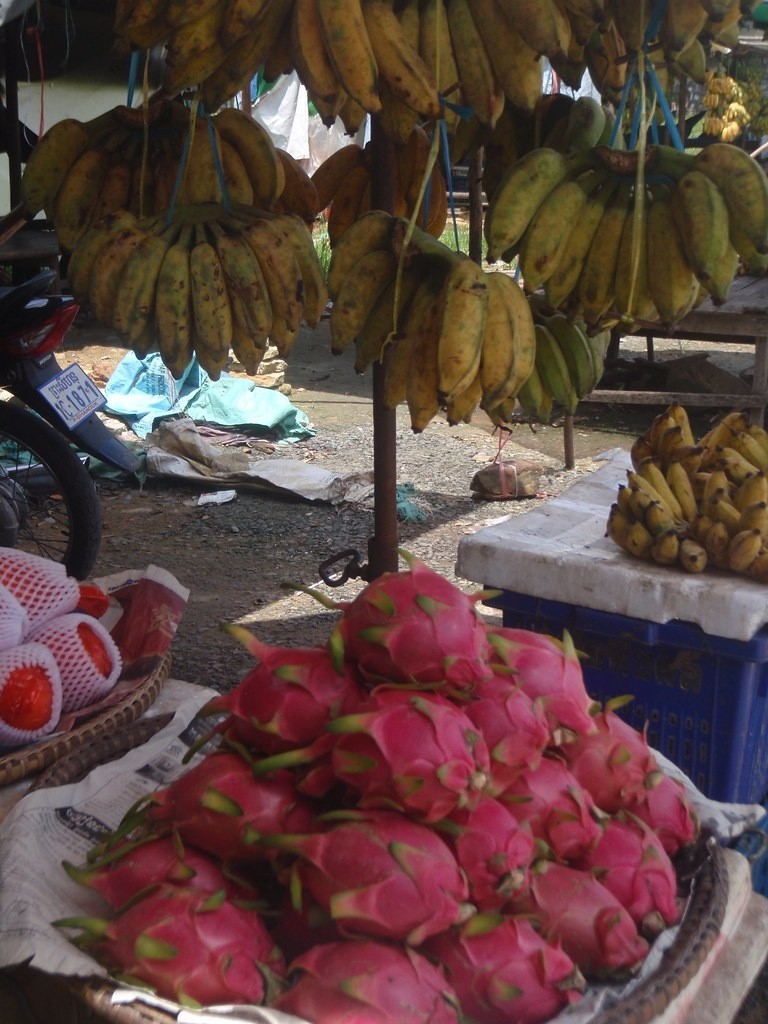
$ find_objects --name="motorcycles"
[1,267,140,588]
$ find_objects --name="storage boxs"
[482,585,768,899]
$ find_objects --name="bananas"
[25,0,768,582]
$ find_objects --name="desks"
[551,270,768,428]
